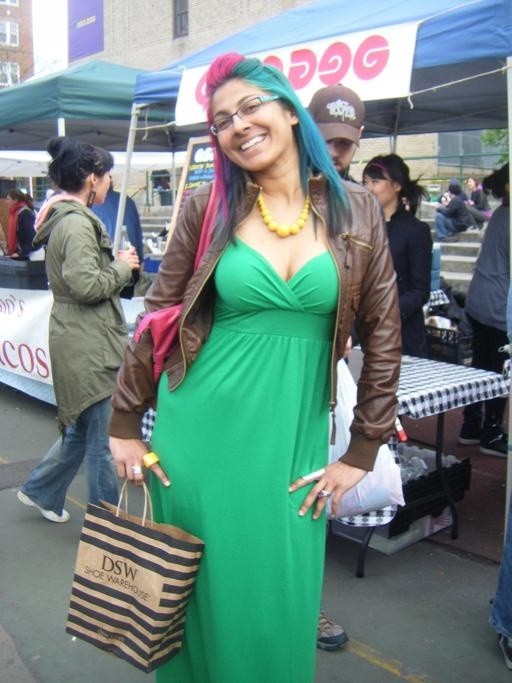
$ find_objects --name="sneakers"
[459,425,507,458]
[17,489,70,523]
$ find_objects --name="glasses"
[210,93,283,136]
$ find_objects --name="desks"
[139,343,510,578]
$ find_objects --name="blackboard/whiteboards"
[163,132,214,256]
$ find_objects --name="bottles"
[113,224,131,263]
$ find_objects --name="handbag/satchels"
[316,613,347,649]
[134,304,184,386]
[67,477,205,674]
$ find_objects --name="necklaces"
[256,193,310,238]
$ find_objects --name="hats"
[308,82,364,146]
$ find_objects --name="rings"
[145,452,159,469]
[131,464,142,475]
[318,489,333,497]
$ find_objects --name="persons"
[307,84,366,654]
[17,133,141,521]
[108,51,401,683]
[6,187,45,261]
[90,176,144,299]
[361,156,512,673]
